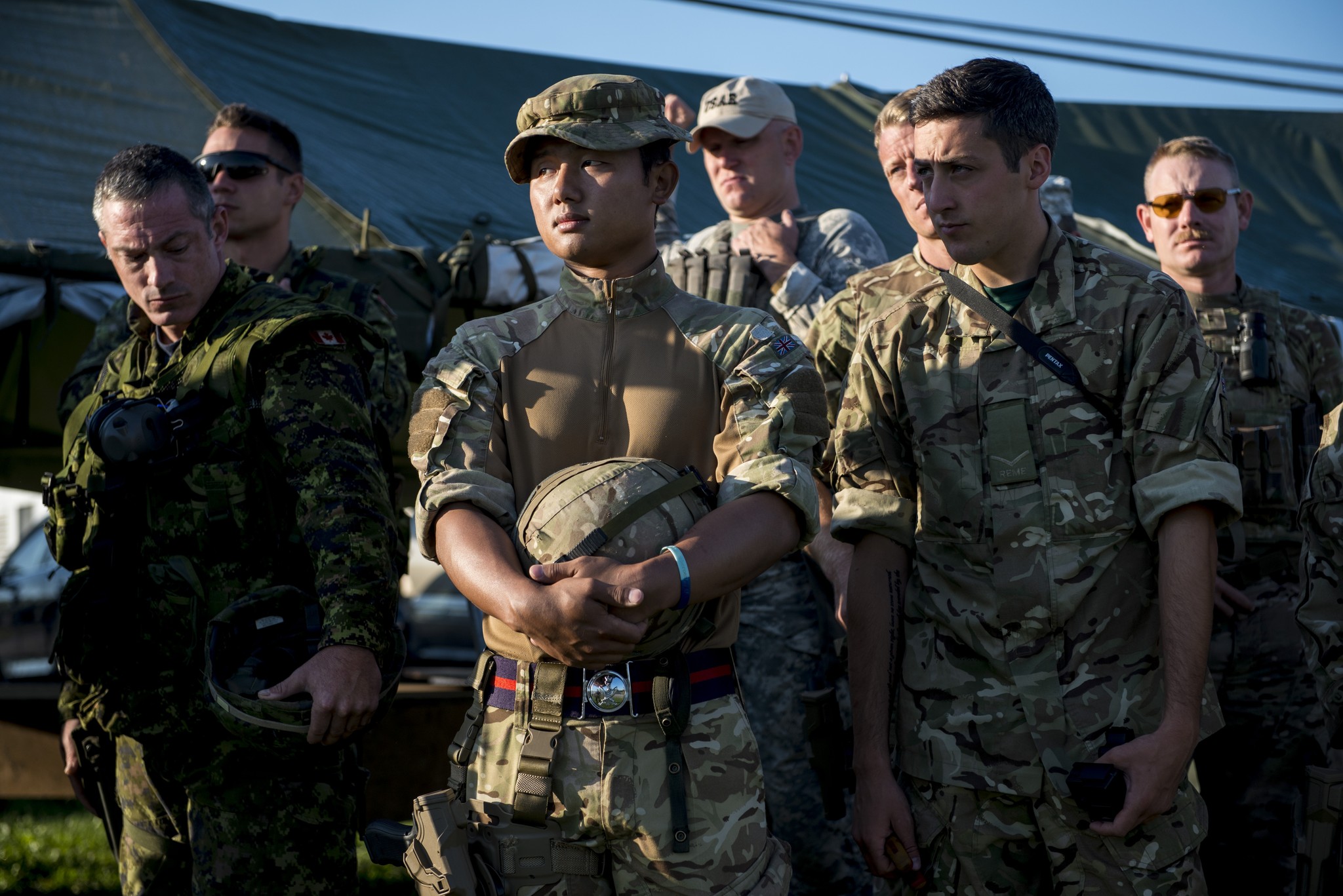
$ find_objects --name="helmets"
[201,586,407,752]
[510,456,717,660]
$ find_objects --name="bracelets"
[658,542,694,617]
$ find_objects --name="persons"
[773,77,1112,426]
[800,52,1254,895]
[406,67,840,896]
[41,141,413,896]
[36,101,458,419]
[1135,139,1343,896]
[639,79,897,896]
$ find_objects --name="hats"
[504,71,694,185]
[685,74,798,155]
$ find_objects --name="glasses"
[1144,187,1241,218]
[190,150,294,184]
